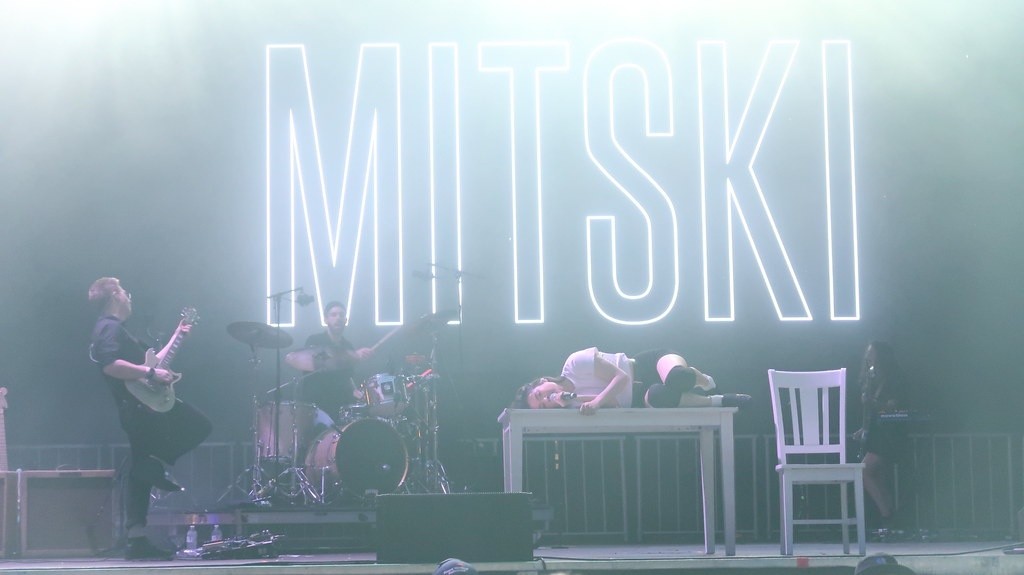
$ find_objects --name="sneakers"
[721,392,753,408]
[702,388,721,396]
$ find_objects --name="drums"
[255,398,318,464]
[360,372,409,417]
[304,414,410,509]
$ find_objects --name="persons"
[510,345,752,416]
[852,340,905,523]
[305,301,374,419]
[86,277,213,561]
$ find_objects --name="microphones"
[550,392,577,402]
[296,294,314,304]
[412,270,435,279]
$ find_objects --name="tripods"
[402,262,485,492]
[208,286,329,511]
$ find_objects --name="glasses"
[115,292,131,301]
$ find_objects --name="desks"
[498,406,740,556]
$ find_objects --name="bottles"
[186,525,198,550]
[211,525,223,542]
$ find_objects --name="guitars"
[124,305,202,414]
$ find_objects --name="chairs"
[768,367,867,556]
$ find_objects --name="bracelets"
[146,368,155,378]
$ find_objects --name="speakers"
[375,491,534,564]
[16,470,118,558]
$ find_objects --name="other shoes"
[134,457,182,491]
[123,537,175,561]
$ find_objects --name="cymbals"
[401,309,458,348]
[226,321,293,349]
[286,345,342,372]
